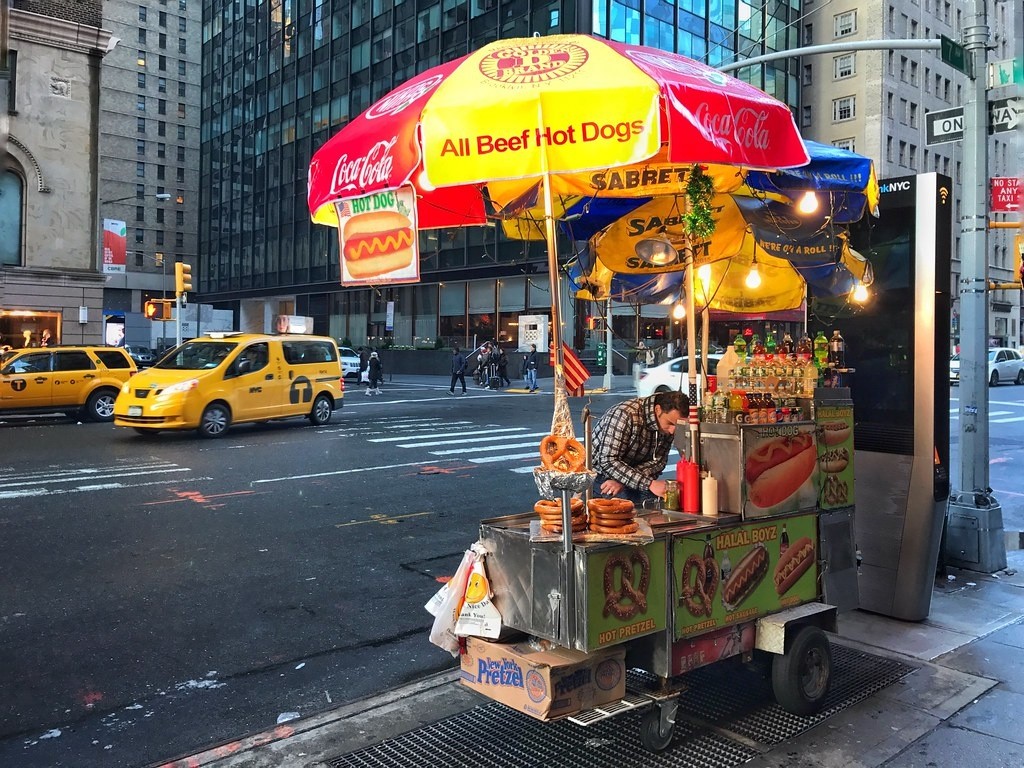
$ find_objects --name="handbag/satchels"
[360,366,369,381]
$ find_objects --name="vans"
[113,333,345,439]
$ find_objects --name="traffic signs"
[925,93,1024,147]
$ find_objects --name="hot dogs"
[817,420,852,472]
[722,546,769,610]
[745,432,817,509]
[774,537,816,595]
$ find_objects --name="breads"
[540,435,586,472]
[823,475,849,505]
[678,554,719,618]
[534,497,639,534]
[601,549,651,622]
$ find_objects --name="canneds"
[775,407,805,422]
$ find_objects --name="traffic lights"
[641,322,666,339]
[582,315,600,331]
[144,300,172,319]
[174,262,192,297]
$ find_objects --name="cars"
[715,348,724,354]
[637,353,752,400]
[949,346,1024,387]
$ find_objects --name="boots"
[365,388,372,396]
[375,387,382,395]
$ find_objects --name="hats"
[371,352,378,357]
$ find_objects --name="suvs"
[0,343,139,423]
[303,346,361,378]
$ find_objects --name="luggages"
[490,364,499,390]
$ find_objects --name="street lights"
[126,250,166,350]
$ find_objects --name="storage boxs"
[459,635,626,722]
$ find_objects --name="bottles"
[665,478,680,511]
[700,331,834,425]
[676,455,718,516]
[829,330,845,367]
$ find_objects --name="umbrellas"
[307,33,812,553]
[559,139,881,457]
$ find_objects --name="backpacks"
[459,355,468,369]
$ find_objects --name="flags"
[550,324,591,395]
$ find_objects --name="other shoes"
[529,390,534,392]
[525,386,529,390]
[507,382,511,386]
[534,386,539,390]
[460,392,467,396]
[446,391,454,396]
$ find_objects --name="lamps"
[635,196,684,266]
[78,305,88,324]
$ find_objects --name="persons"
[39,328,54,348]
[356,347,370,386]
[18,330,36,348]
[521,344,539,393]
[582,392,689,505]
[477,342,511,387]
[372,347,385,385]
[365,352,383,396]
[106,323,124,347]
[271,315,289,333]
[445,347,467,396]
[246,349,264,371]
